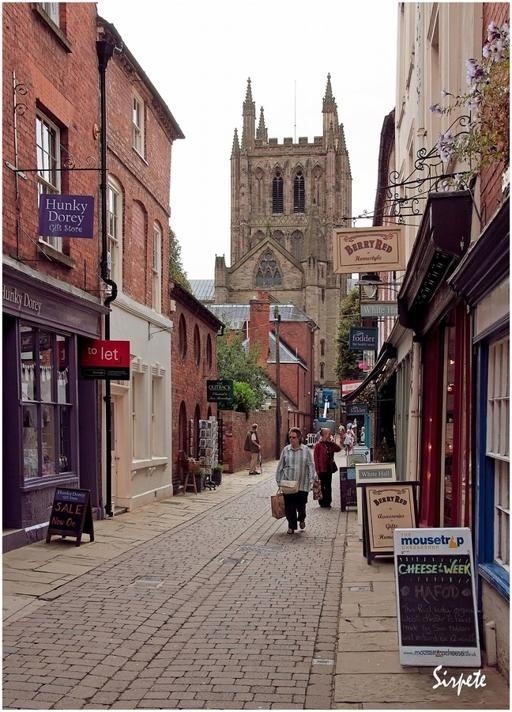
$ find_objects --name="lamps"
[355,272,405,298]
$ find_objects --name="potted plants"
[211,464,224,485]
[186,466,204,492]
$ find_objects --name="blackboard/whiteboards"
[48,487,89,537]
[83,490,94,534]
[397,554,479,647]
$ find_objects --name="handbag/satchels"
[279,480,299,495]
[313,479,322,501]
[271,494,286,519]
[330,462,338,473]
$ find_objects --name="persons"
[314,426,342,509]
[246,422,262,475]
[275,427,319,534]
[314,423,365,457]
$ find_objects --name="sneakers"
[287,529,293,534]
[300,521,306,529]
[249,471,261,475]
